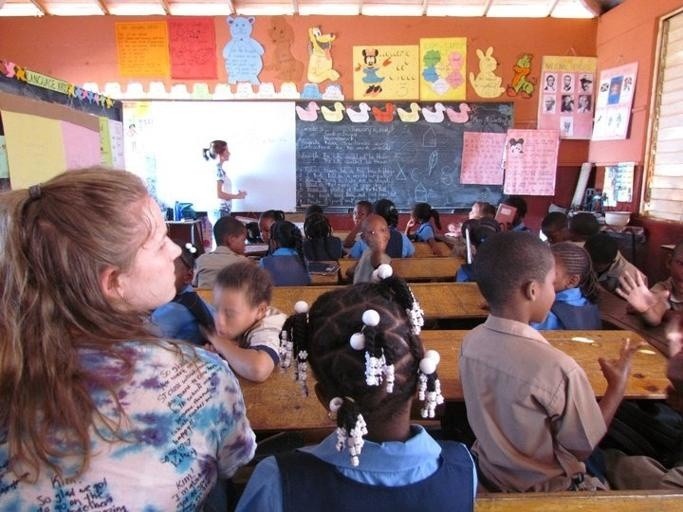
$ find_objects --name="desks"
[193,228,682,511]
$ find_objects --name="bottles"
[173,200,181,222]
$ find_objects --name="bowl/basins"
[605,210,633,227]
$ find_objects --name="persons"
[203,140,247,252]
[562,95,574,112]
[578,76,593,93]
[544,75,556,90]
[458,232,642,494]
[190,198,447,290]
[561,75,573,93]
[588,239,683,495]
[577,95,590,113]
[456,196,648,331]
[235,266,478,512]
[1,166,258,511]
[544,96,556,113]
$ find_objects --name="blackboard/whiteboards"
[295,99,513,213]
